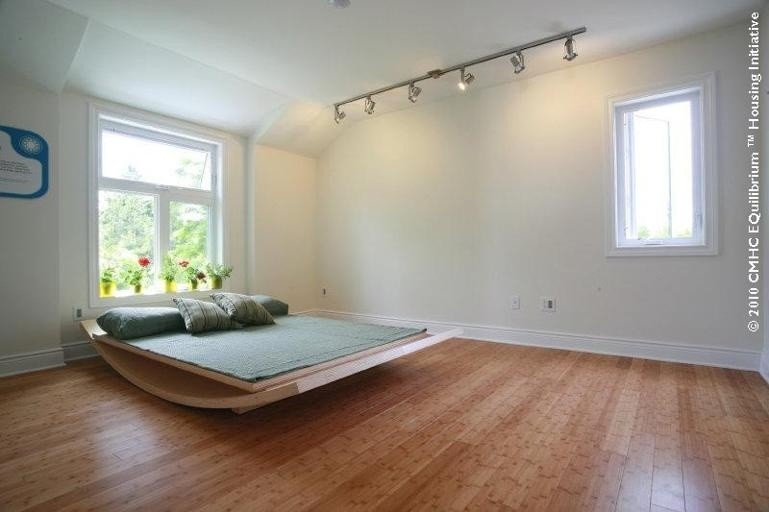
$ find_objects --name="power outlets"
[509,295,557,313]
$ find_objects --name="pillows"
[95,292,291,340]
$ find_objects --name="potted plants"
[99,266,119,298]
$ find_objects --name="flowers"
[123,256,233,285]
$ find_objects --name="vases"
[132,276,224,294]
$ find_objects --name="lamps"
[333,25,588,126]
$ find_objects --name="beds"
[78,291,463,416]
